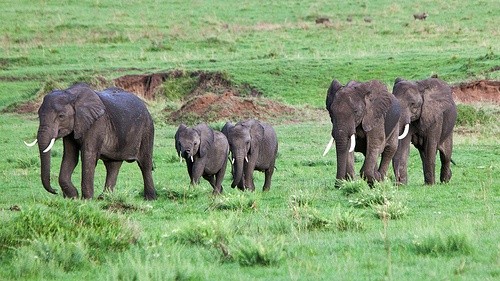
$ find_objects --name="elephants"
[23,82,157,201]
[322,80,405,189]
[220,118,279,192]
[392,73,457,185]
[174,123,231,193]
